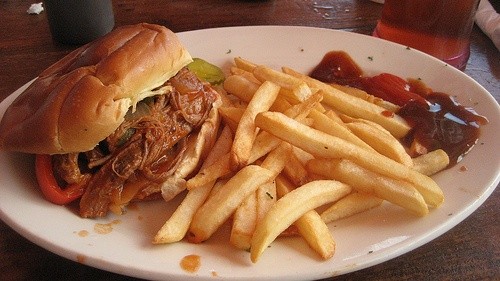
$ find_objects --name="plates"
[0,25,500,281]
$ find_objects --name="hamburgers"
[1,22,222,220]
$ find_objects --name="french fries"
[151,56,449,262]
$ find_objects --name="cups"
[373,0,481,69]
[43,0,114,49]
